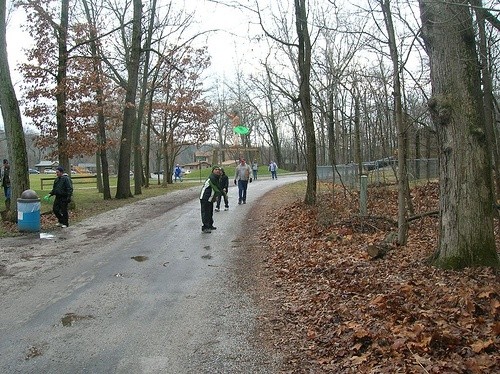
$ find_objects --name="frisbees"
[234,125,249,135]
[44,196,53,204]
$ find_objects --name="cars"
[28,168,40,174]
[44,168,57,174]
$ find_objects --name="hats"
[211,165,219,173]
[219,166,224,171]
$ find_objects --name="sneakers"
[224,207,228,211]
[61,225,67,227]
[203,228,211,234]
[210,226,216,229]
[56,222,62,226]
[215,207,219,212]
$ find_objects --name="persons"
[174,166,183,182]
[199,165,228,233]
[268,160,278,180]
[1,158,11,204]
[251,158,258,180]
[215,166,229,212]
[234,157,252,205]
[47,166,73,228]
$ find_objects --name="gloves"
[234,180,236,184]
[250,178,252,183]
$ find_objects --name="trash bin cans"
[17,190,41,232]
